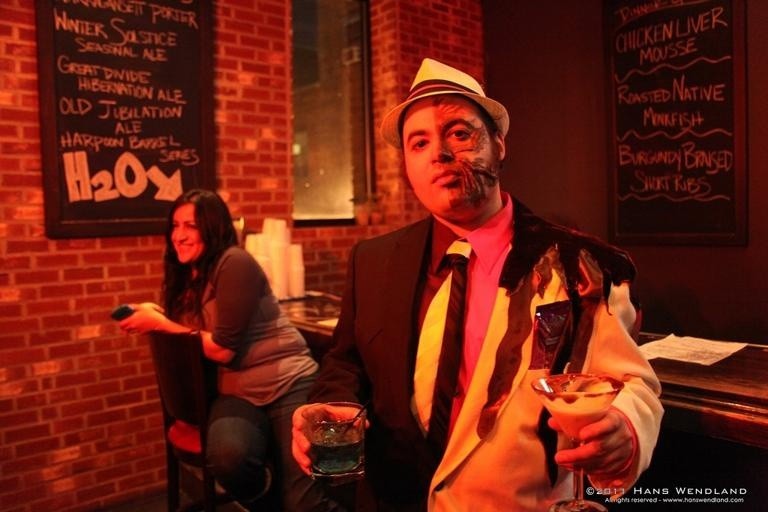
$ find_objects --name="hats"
[379,57,510,151]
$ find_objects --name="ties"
[413,240,472,474]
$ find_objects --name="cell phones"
[111,303,135,323]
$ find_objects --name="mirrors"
[292,2,376,224]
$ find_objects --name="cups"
[300,401,368,487]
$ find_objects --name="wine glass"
[530,372,626,511]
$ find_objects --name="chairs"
[149,328,245,512]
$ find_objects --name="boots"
[240,468,283,511]
